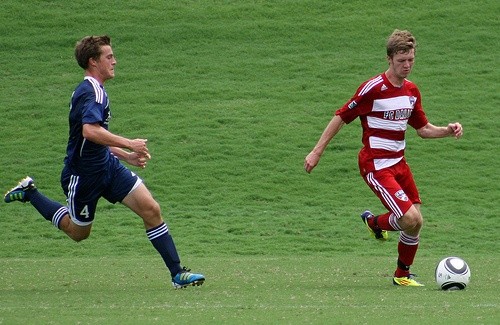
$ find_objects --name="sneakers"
[3,175,36,204]
[171,266,206,289]
[361,211,388,241]
[392,273,426,287]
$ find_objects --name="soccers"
[434,256,471,291]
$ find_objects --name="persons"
[4,34,206,290]
[304,29,463,286]
